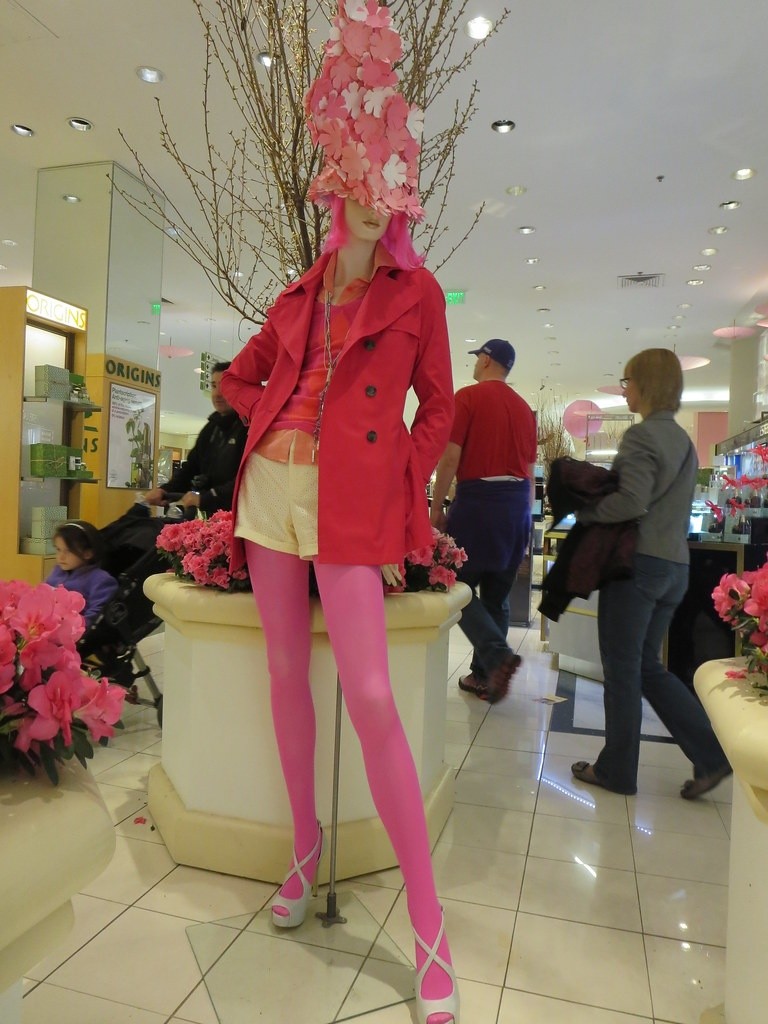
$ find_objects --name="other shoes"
[571,761,638,795]
[680,757,733,798]
[458,672,489,696]
[486,650,522,699]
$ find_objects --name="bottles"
[165,503,183,519]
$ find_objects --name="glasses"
[619,377,635,389]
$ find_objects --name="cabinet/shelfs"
[0,286,102,585]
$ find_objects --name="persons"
[571,349,733,802]
[221,0,462,1024]
[146,361,249,517]
[428,339,536,702]
[40,518,120,635]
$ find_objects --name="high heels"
[413,907,458,1024]
[270,819,325,929]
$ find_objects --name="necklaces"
[311,280,359,463]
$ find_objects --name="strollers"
[0,493,199,775]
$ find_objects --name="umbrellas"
[674,348,710,370]
[714,305,768,340]
[563,399,602,439]
[596,386,628,397]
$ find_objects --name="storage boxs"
[30,443,67,477]
[35,364,69,400]
[20,506,67,555]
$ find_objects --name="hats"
[467,339,516,370]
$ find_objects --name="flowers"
[0,579,129,786]
[711,552,768,694]
[156,508,468,594]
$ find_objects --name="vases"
[1,756,116,1023]
[143,574,472,887]
[693,656,767,1024]
